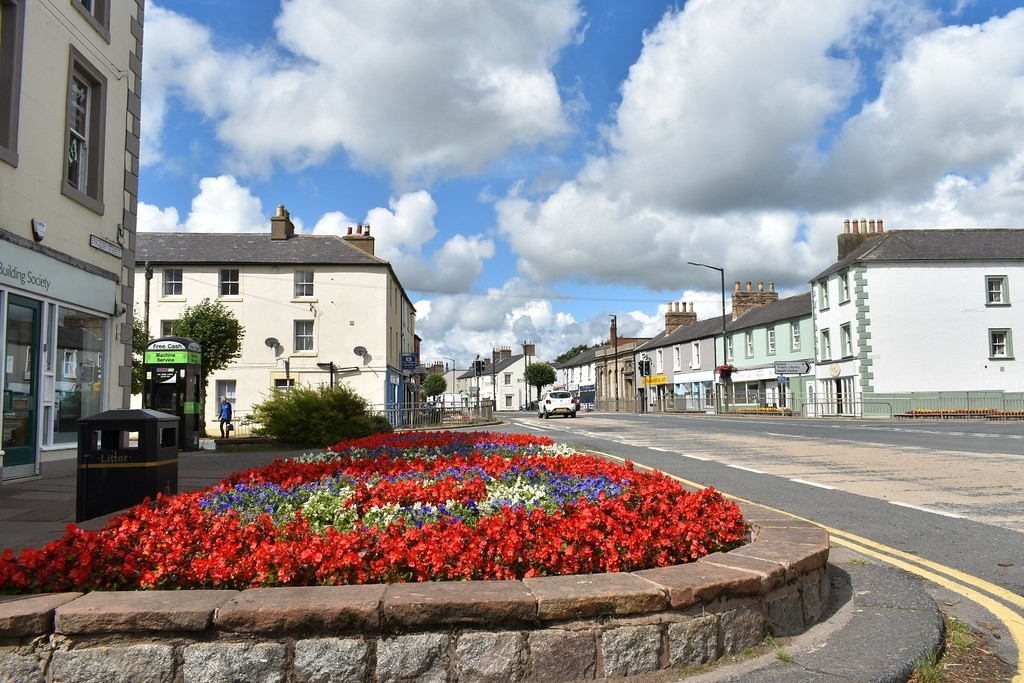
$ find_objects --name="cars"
[519,400,538,411]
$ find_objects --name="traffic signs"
[773,360,811,374]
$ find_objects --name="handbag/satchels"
[226,424,234,431]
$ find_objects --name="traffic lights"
[644,361,650,376]
[475,360,482,377]
[638,360,644,377]
[481,361,486,373]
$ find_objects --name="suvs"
[538,391,577,419]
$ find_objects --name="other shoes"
[220,437,224,439]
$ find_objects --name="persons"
[217,396,231,439]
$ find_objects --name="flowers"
[715,362,737,383]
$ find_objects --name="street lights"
[443,357,455,414]
[608,314,619,411]
[687,261,727,367]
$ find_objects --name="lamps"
[352,344,368,358]
[262,336,280,350]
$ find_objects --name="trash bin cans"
[76,409,180,521]
